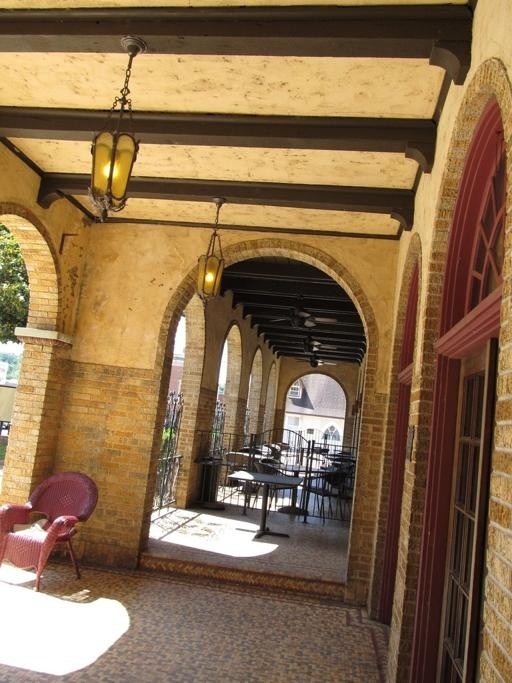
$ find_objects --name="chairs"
[0,472,99,592]
[193,442,356,538]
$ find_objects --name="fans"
[269,293,338,367]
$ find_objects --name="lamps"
[88,34,148,223]
[197,196,226,308]
